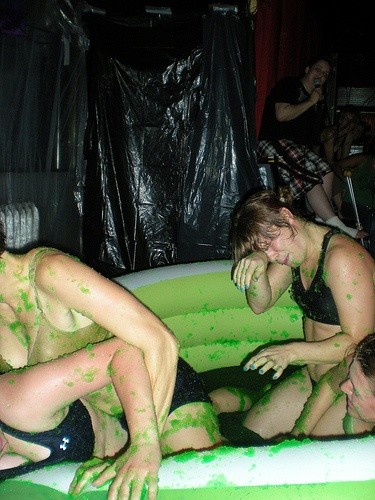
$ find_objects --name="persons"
[311,104,372,218]
[275,332,375,443]
[256,57,369,239]
[337,121,371,160]
[228,188,375,444]
[0,204,222,500]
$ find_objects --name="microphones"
[314,79,321,108]
